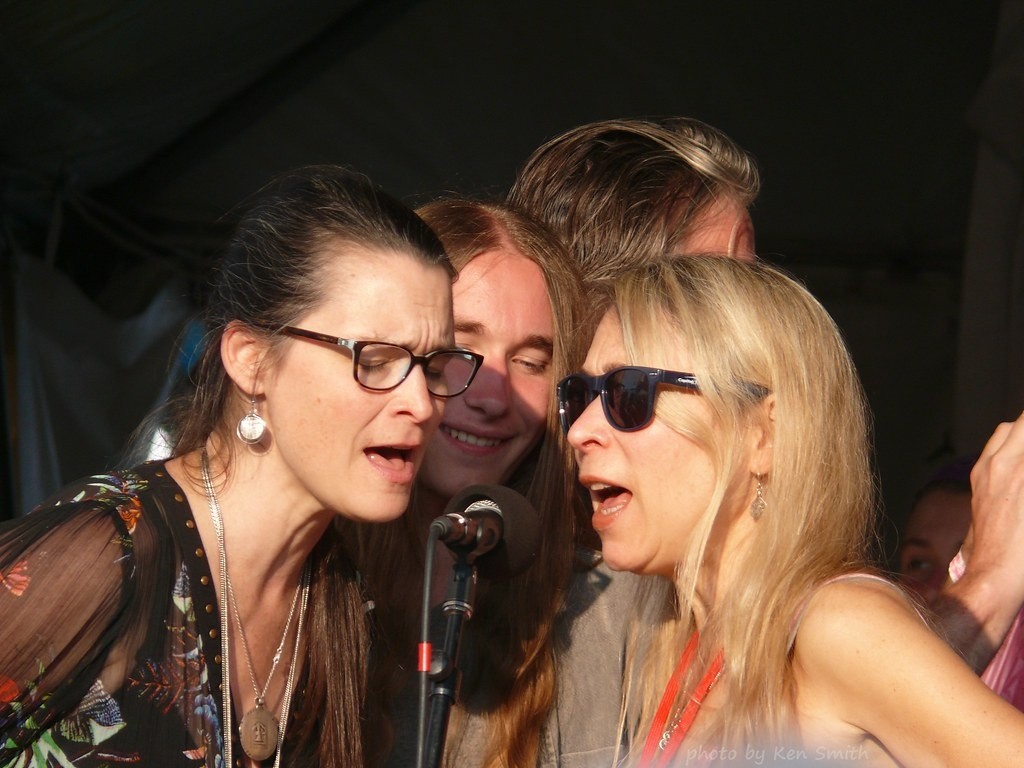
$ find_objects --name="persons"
[899,478,972,604]
[331,201,590,768]
[1,164,485,767]
[557,255,1023,768]
[505,119,1023,681]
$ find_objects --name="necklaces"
[201,444,313,768]
[638,630,725,768]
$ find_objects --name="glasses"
[259,321,486,401]
[555,366,773,437]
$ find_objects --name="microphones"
[431,483,543,581]
